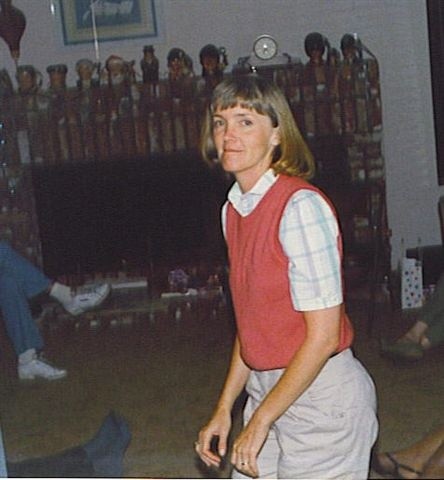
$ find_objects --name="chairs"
[335,184,396,336]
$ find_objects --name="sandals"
[368,452,423,480]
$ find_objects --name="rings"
[241,462,248,465]
[195,442,200,444]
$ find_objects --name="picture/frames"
[50,0,166,53]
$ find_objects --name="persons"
[340,33,362,64]
[200,44,228,74]
[195,74,380,480]
[0,241,112,380]
[304,33,337,64]
[15,45,161,95]
[167,49,192,79]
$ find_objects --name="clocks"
[252,34,278,60]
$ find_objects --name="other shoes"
[380,341,423,359]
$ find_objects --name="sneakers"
[17,359,66,380]
[64,283,110,316]
